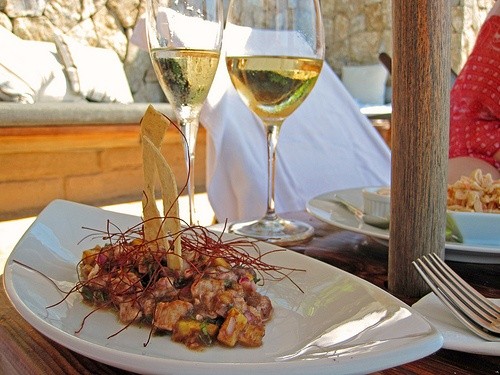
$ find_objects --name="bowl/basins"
[445,208,500,247]
[362,187,390,229]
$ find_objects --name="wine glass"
[144,0,224,227]
[223,0,326,247]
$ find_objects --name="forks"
[411,252,500,342]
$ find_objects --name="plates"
[1,197,444,375]
[305,186,500,264]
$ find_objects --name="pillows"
[341,65,389,107]
[0,26,87,105]
[55,33,134,104]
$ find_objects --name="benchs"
[0,103,393,225]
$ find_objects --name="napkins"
[412,287,500,355]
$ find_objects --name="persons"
[446,0,500,186]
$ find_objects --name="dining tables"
[0,212,500,375]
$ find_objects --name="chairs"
[158,12,391,225]
